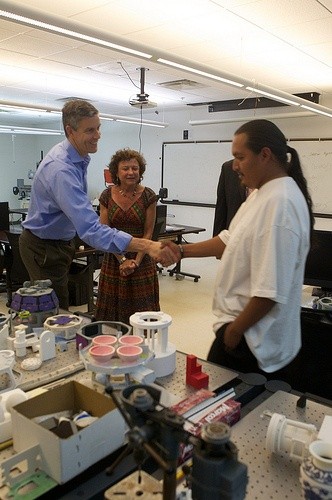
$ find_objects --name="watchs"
[118,257,126,263]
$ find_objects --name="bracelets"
[133,260,138,269]
[178,244,184,259]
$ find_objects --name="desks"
[0,292,332,500]
[0,202,206,319]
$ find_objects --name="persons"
[93,149,161,338]
[19,99,181,311]
[149,118,315,386]
[213,159,258,260]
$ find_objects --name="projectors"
[129,97,158,109]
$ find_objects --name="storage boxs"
[11,380,126,484]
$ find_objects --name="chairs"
[0,230,13,308]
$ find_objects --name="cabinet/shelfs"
[270,291,332,401]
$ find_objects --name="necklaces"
[117,184,138,200]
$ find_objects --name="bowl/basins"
[93,335,117,346]
[118,336,143,346]
[117,345,142,360]
[89,345,115,361]
